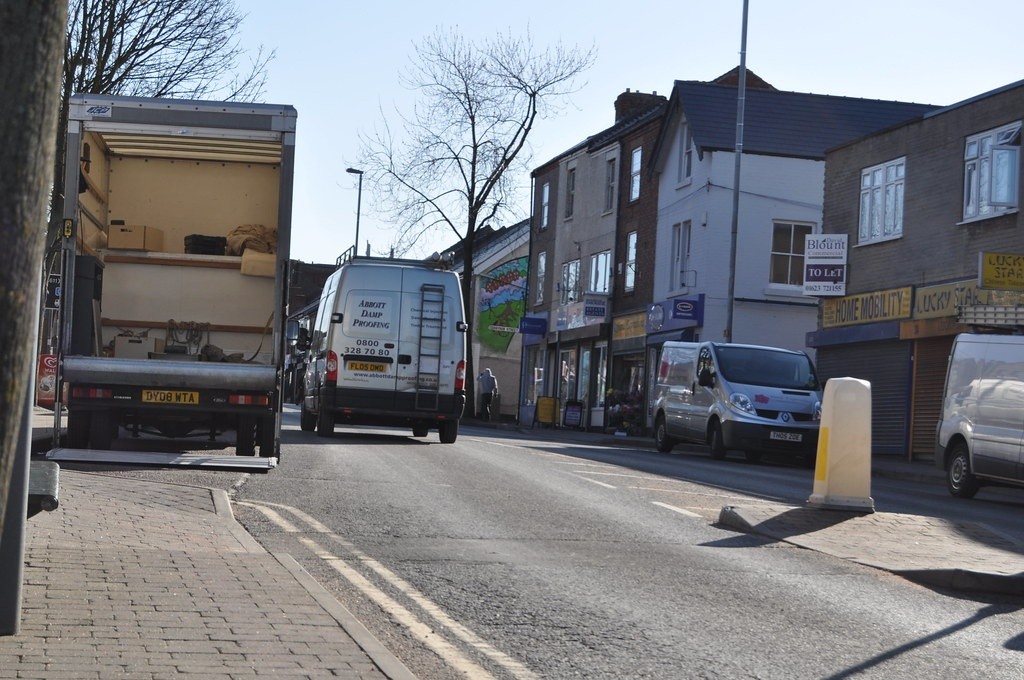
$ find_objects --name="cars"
[284,351,306,405]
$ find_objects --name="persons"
[476,368,498,423]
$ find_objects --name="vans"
[650,341,823,461]
[296,246,469,443]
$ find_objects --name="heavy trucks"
[46,94,300,469]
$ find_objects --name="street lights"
[346,168,364,252]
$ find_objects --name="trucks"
[934,332,1024,498]
